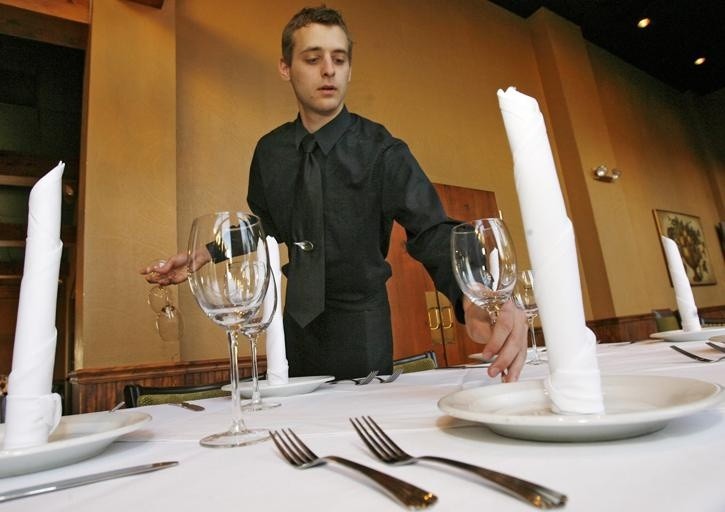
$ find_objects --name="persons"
[136,1,531,386]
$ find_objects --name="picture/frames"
[652,207,717,287]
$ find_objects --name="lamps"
[590,162,623,183]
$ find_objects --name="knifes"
[0,459,185,507]
[168,401,204,412]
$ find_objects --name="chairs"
[121,372,265,408]
[391,346,439,373]
[651,307,681,334]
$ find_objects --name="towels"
[5,153,74,451]
[498,84,606,416]
[659,233,703,331]
[256,233,293,385]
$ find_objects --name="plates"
[433,373,725,442]
[0,406,153,478]
[646,326,723,343]
[219,375,333,397]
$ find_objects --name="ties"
[286,137,324,328]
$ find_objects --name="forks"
[263,427,437,512]
[325,368,378,389]
[663,339,724,363]
[375,364,409,387]
[350,413,569,512]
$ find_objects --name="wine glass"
[181,207,268,452]
[509,271,547,365]
[220,256,283,414]
[449,218,525,381]
[145,261,184,344]
